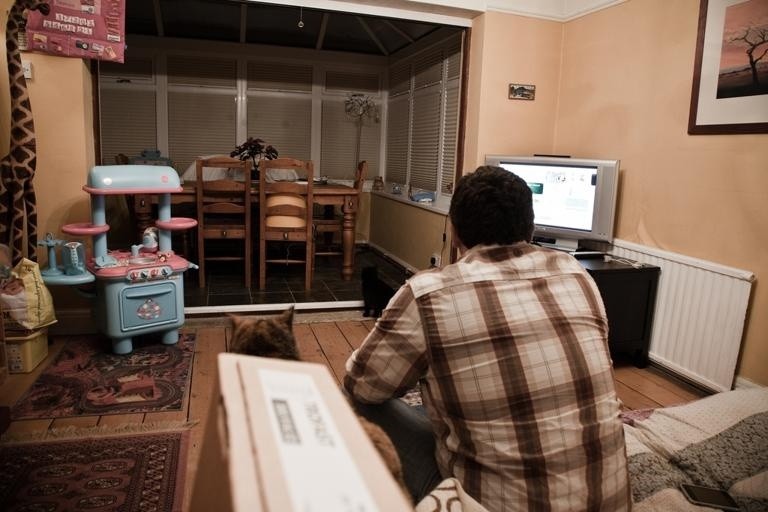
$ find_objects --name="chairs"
[116,149,368,291]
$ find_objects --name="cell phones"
[680,483,740,511]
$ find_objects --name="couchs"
[195,348,768,511]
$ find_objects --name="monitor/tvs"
[484,154,620,253]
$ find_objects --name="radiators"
[576,231,757,395]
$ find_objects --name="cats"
[361,265,396,318]
[224,305,415,507]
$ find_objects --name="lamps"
[342,93,380,178]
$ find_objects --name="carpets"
[0,331,198,511]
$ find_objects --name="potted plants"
[230,137,280,177]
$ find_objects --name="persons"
[344,166,635,512]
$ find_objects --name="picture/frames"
[686,2,768,134]
